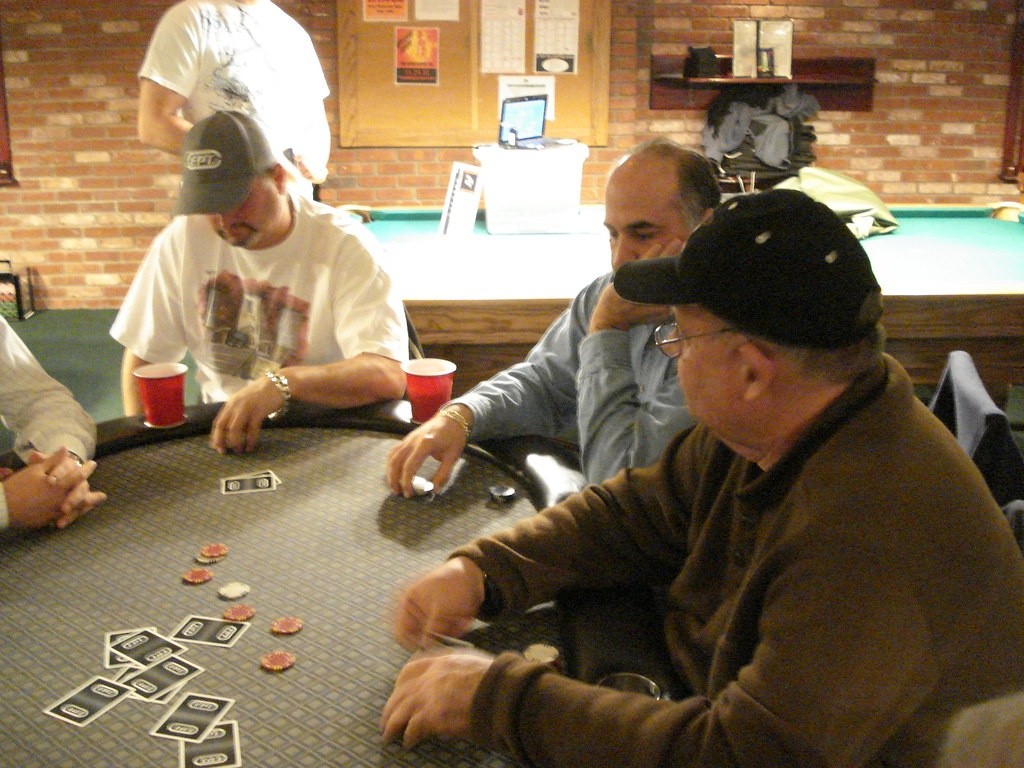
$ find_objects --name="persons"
[0,312,107,531]
[388,136,722,499]
[109,111,410,455]
[137,0,331,202]
[378,189,1024,768]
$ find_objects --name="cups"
[133,362,188,425]
[400,358,457,422]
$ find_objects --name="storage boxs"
[472,139,588,236]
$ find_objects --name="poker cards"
[405,631,476,668]
[217,468,284,496]
[41,611,252,768]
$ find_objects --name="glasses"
[653,320,738,361]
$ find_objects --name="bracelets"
[438,409,470,442]
[266,370,291,421]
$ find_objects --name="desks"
[0,401,677,768]
[340,200,1024,424]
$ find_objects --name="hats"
[613,188,883,350]
[174,110,278,214]
